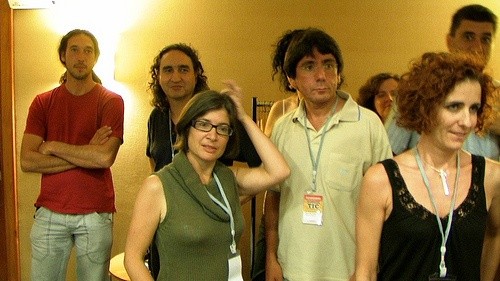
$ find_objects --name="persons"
[445,5,500,131]
[266,28,392,281]
[145,40,264,206]
[263,29,299,156]
[19,27,123,280]
[355,51,499,280]
[358,74,406,120]
[124,79,292,281]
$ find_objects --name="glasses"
[191,119,233,136]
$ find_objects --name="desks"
[109,251,150,281]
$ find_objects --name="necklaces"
[423,157,453,197]
